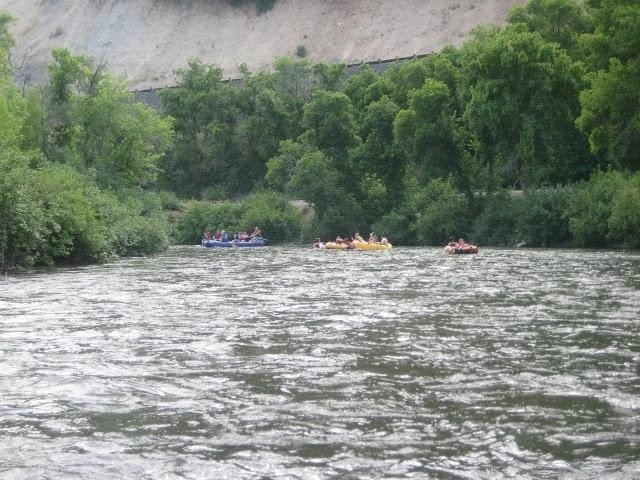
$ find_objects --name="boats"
[202,237,268,247]
[444,244,478,254]
[325,240,392,250]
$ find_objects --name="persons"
[448,235,469,247]
[200,226,262,241]
[314,232,389,252]
